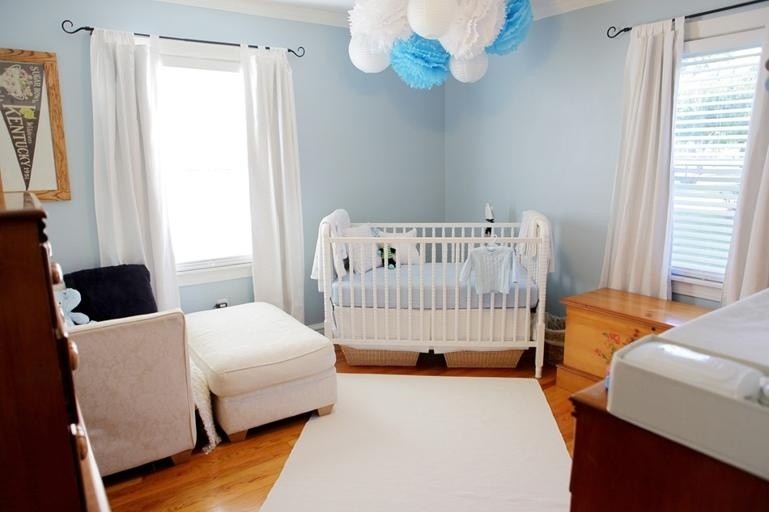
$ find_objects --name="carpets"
[258,373,573,512]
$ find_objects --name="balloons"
[348,31,391,75]
[449,51,488,83]
[407,0,458,42]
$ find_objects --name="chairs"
[53,262,197,479]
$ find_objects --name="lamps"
[346,0,533,91]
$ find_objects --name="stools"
[183,301,338,443]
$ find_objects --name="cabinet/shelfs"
[1,209,89,512]
[566,376,767,511]
[556,287,716,393]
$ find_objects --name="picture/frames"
[0,47,72,202]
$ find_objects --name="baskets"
[341,347,421,367]
[545,311,566,367]
[444,350,524,368]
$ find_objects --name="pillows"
[339,222,421,275]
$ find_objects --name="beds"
[321,209,550,380]
